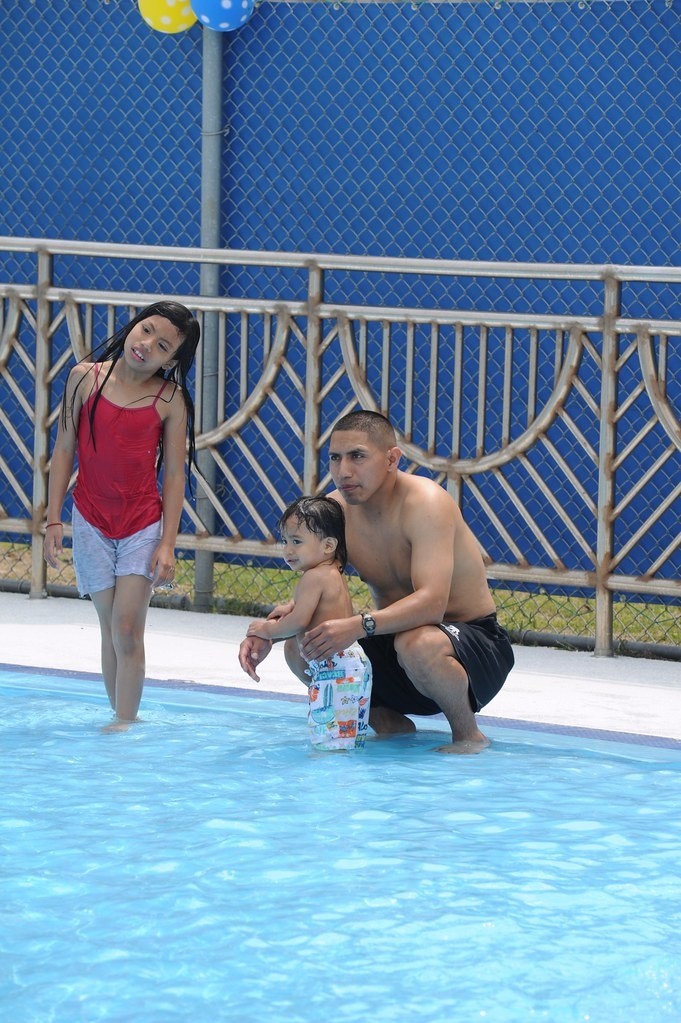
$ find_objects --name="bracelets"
[45,523,63,529]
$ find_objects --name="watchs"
[361,612,377,639]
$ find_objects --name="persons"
[238,410,515,755]
[43,300,210,721]
[246,496,373,750]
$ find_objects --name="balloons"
[137,0,255,34]
